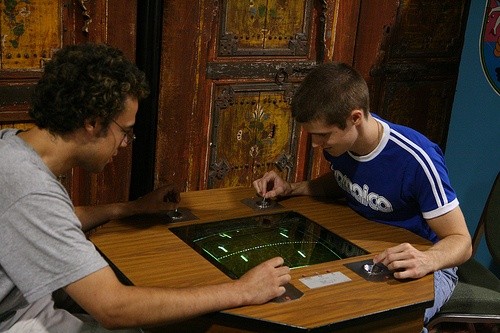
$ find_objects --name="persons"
[253,61,472,333]
[0,41,292,333]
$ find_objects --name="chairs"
[423,171,500,333]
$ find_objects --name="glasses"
[110,117,137,146]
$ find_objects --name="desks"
[85,185,435,333]
